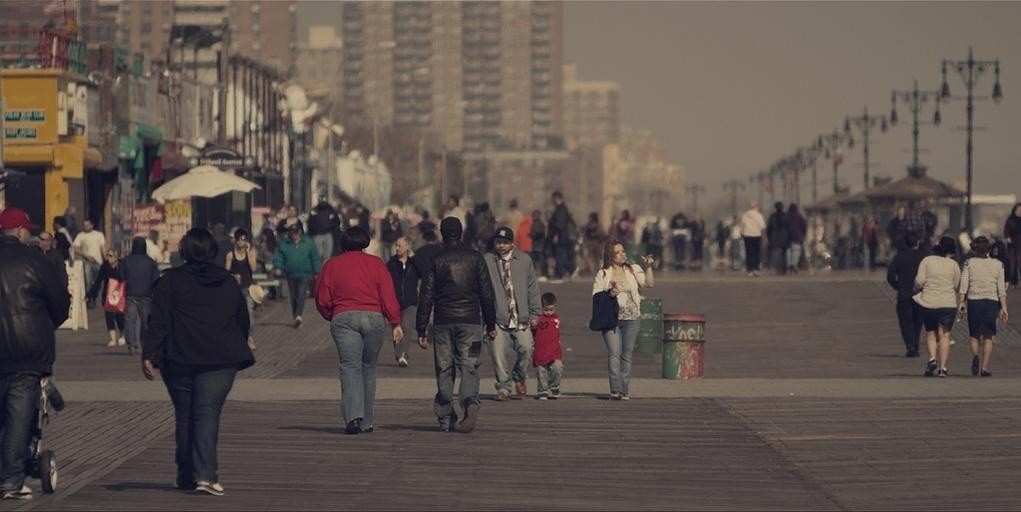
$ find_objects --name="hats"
[285,224,300,232]
[248,284,265,305]
[491,225,515,244]
[0,206,42,233]
[930,235,959,251]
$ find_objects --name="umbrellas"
[150,166,262,232]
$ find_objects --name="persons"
[140,229,253,496]
[1,205,74,499]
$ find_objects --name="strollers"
[27,377,67,496]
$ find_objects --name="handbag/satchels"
[100,275,129,315]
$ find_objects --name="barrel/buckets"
[633,296,664,353]
[661,310,706,379]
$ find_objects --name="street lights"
[325,40,397,204]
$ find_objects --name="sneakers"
[434,406,458,432]
[903,346,996,380]
[345,421,374,435]
[179,478,225,499]
[493,379,562,401]
[610,391,631,402]
[397,356,410,369]
[2,486,33,503]
[105,327,144,358]
[456,398,480,434]
[247,335,257,352]
[292,316,305,330]
